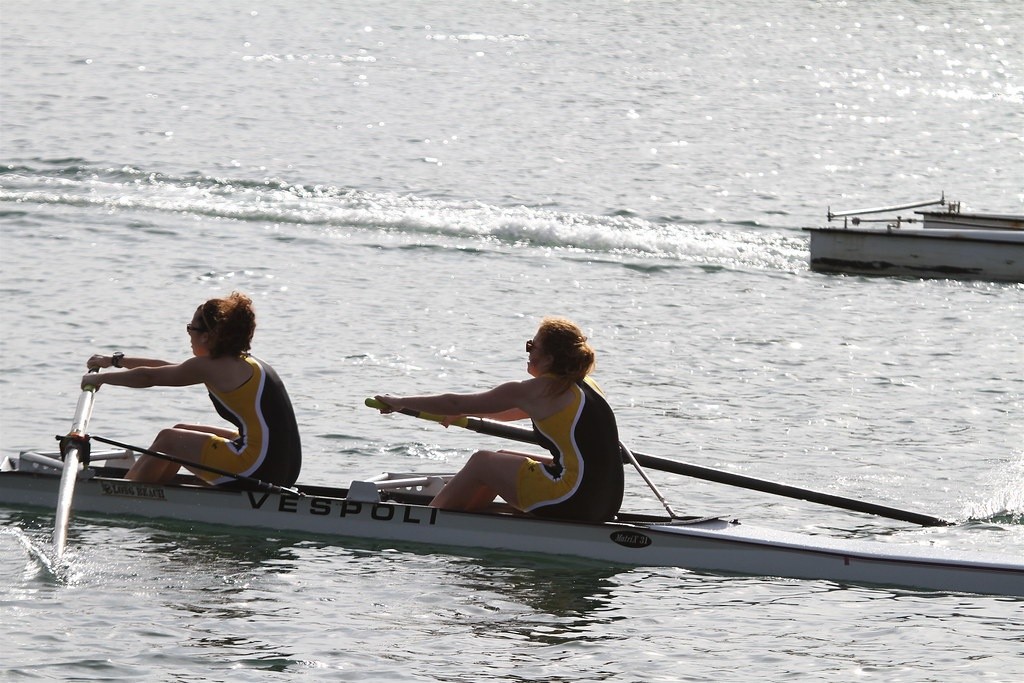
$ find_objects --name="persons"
[81,292,302,493]
[374,321,625,521]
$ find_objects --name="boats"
[1,446,1023,596]
[803,190,1023,287]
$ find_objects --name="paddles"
[364,397,957,527]
[50,366,99,557]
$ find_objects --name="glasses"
[526,339,554,353]
[187,324,209,334]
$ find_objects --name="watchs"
[112,351,125,368]
